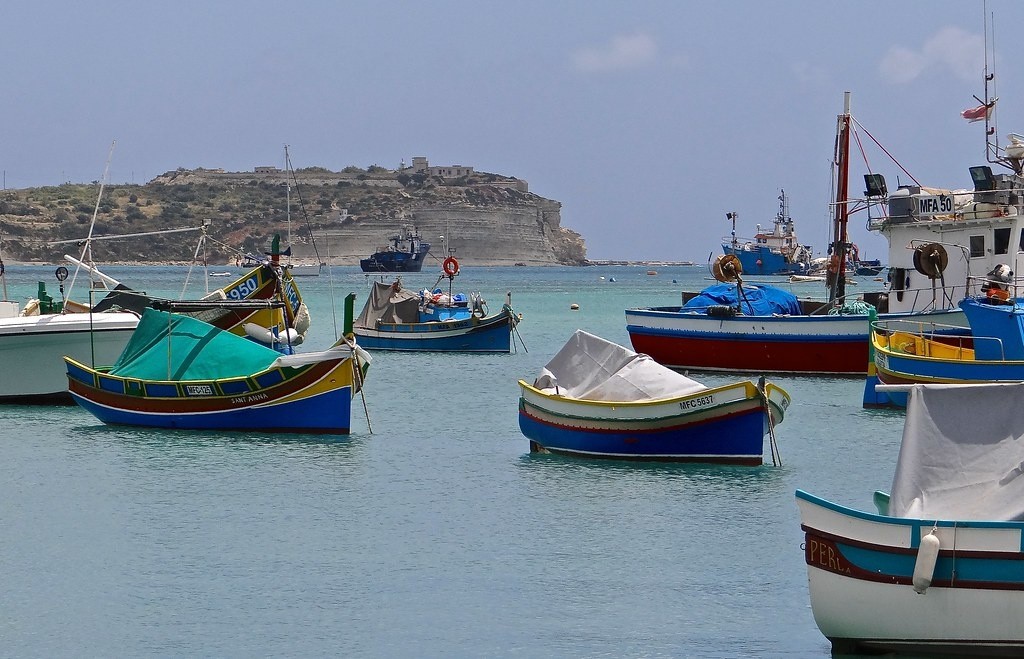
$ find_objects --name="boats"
[515,328,791,466]
[358,222,431,273]
[63,290,374,436]
[0,218,313,400]
[351,211,522,353]
[791,378,1024,659]
[623,1,1024,411]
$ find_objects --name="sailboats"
[263,144,322,276]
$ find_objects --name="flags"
[960,104,993,124]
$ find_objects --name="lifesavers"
[443,257,460,276]
